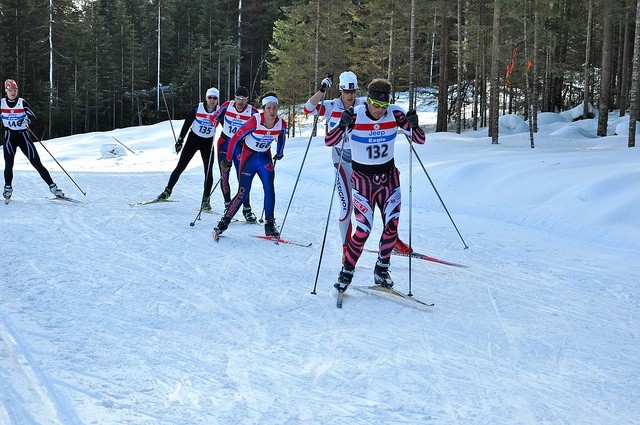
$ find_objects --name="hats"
[206,87,220,99]
[338,71,359,92]
[236,87,249,97]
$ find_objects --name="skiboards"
[213,231,312,247]
[129,198,223,216]
[231,219,265,226]
[5,197,85,205]
[337,285,434,308]
[364,248,470,268]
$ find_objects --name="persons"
[156,87,224,212]
[325,79,425,291]
[303,71,414,264]
[213,92,286,237]
[0,78,66,205]
[210,86,259,222]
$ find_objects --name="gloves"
[273,155,284,160]
[220,160,232,172]
[406,110,419,128]
[340,106,354,128]
[320,77,333,94]
[175,139,183,152]
[22,119,30,129]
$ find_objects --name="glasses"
[369,98,390,109]
[7,89,18,92]
[208,97,218,100]
[343,90,355,95]
[237,97,248,101]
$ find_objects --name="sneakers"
[334,263,355,284]
[393,239,413,254]
[214,222,228,233]
[158,188,172,199]
[2,185,13,199]
[244,212,256,221]
[374,264,394,285]
[49,183,63,197]
[201,198,212,211]
[265,223,279,236]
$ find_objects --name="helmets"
[4,79,18,93]
